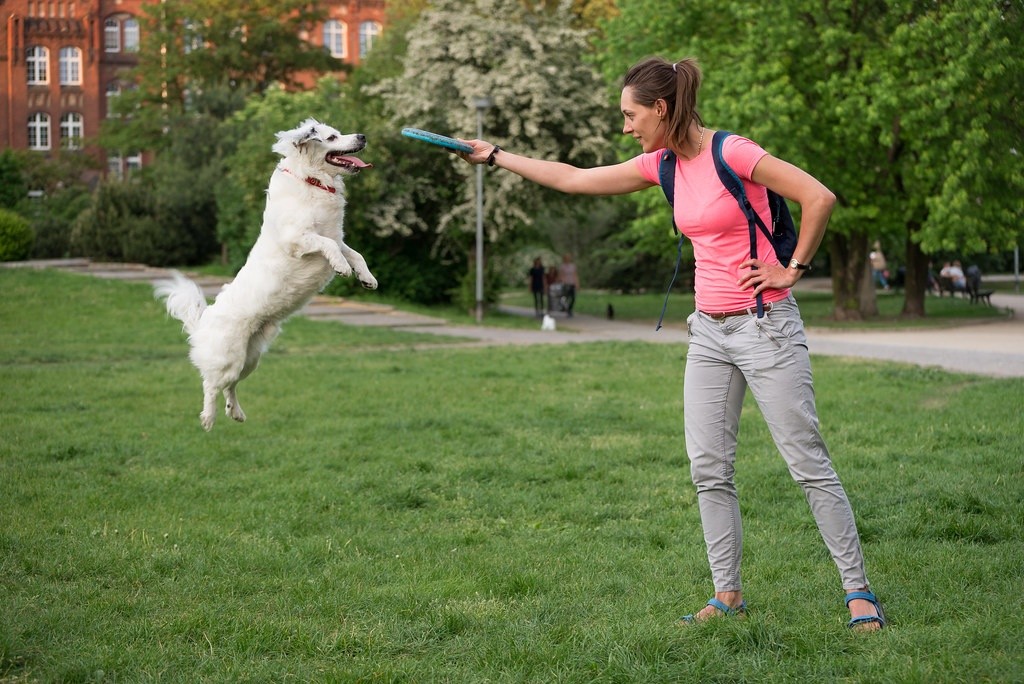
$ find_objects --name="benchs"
[885,267,994,306]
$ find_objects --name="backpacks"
[656,131,798,331]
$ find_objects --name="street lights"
[474,98,488,322]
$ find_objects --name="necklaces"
[697,128,704,155]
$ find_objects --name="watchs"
[790,259,811,271]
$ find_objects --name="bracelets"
[485,145,500,166]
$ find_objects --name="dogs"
[148,118,379,429]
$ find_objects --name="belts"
[699,307,771,320]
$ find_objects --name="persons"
[547,252,579,316]
[927,260,966,297]
[870,241,888,288]
[526,256,547,318]
[446,59,884,634]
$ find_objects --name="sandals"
[679,598,745,626]
[845,587,886,631]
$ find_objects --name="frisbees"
[401,128,475,154]
